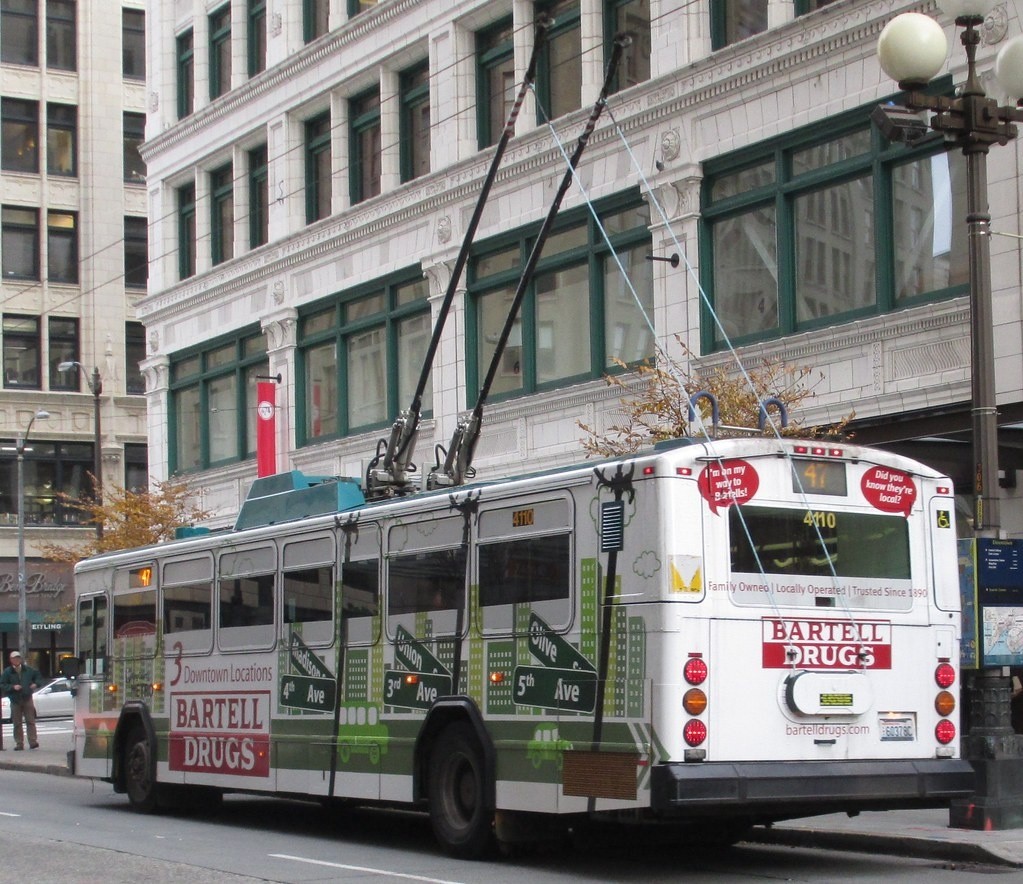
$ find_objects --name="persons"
[0,651,44,751]
[1010,667,1023,734]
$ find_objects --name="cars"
[0,677,76,721]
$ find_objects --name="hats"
[9,651,21,658]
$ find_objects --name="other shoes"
[14,746,24,750]
[30,744,39,749]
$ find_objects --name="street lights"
[15,410,50,664]
[58,360,105,677]
[861,8,1023,884]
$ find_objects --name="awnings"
[0,611,75,632]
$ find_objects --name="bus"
[58,15,976,859]
